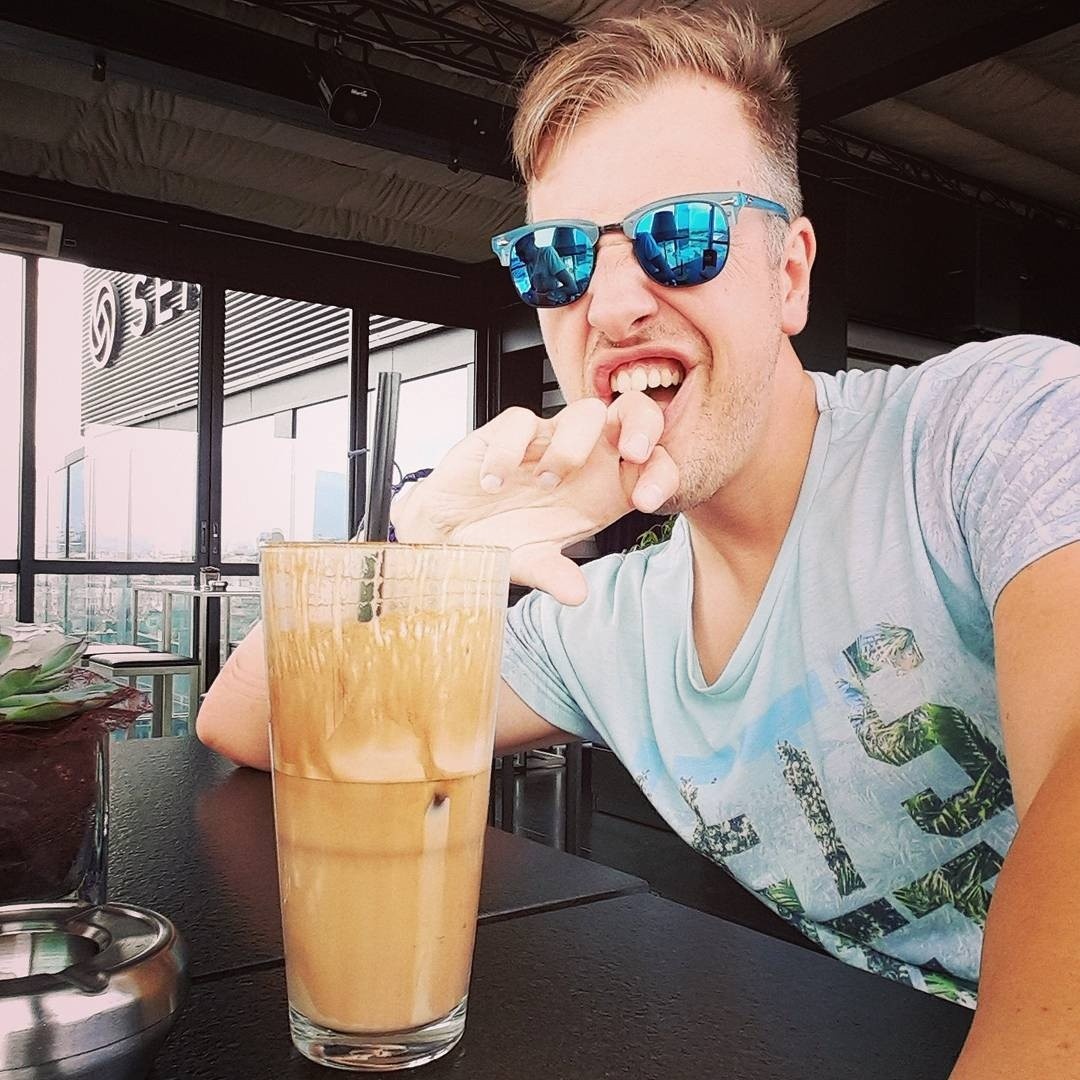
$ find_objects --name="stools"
[88,651,203,738]
[72,644,151,739]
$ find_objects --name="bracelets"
[387,467,433,543]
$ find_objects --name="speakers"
[316,70,382,132]
[948,268,1025,335]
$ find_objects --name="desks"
[104,739,973,1080]
[124,584,260,740]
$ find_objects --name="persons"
[636,230,678,284]
[190,1,1080,1078]
[515,233,581,305]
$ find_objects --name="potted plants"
[0,621,156,904]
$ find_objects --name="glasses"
[516,239,530,256]
[491,192,787,307]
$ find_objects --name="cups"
[200,572,220,591]
[257,539,512,1072]
[0,726,111,905]
